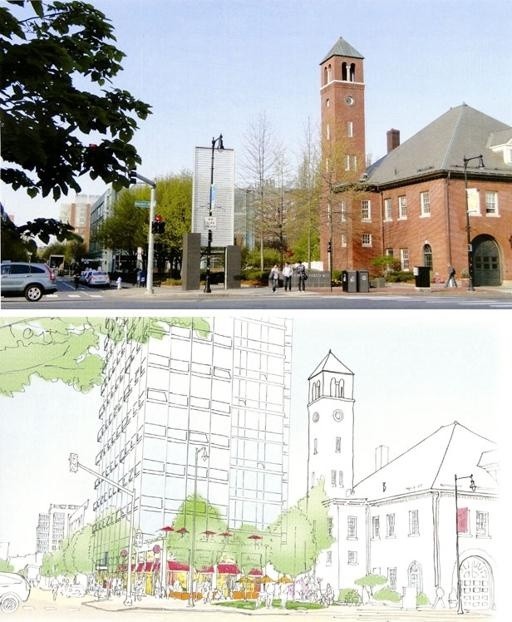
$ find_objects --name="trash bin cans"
[341,270,369,293]
[402,586,417,612]
[136,270,146,288]
[414,266,431,288]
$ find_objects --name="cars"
[0,571,29,612]
[66,584,84,598]
[79,270,111,287]
[1,262,58,302]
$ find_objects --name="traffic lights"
[152,221,165,233]
[156,214,161,220]
[69,453,78,471]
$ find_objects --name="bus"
[49,255,66,275]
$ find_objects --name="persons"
[132,267,146,289]
[267,263,281,294]
[295,260,305,293]
[281,262,294,293]
[71,265,81,290]
[443,262,457,288]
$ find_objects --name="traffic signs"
[135,201,150,208]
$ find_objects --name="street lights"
[463,155,485,290]
[454,474,475,614]
[187,448,208,605]
[204,133,224,293]
